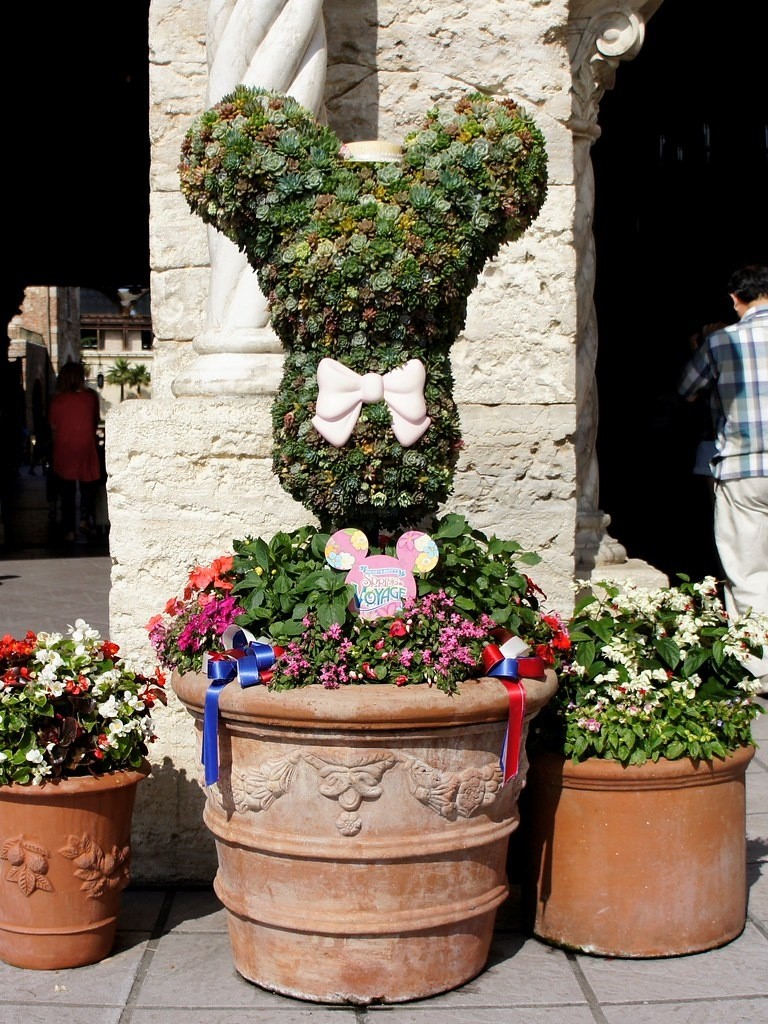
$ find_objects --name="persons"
[17,362,101,541]
[678,266,768,697]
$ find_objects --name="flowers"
[144,512,571,696]
[0,618,168,788]
[530,573,768,765]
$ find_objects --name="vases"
[171,666,559,1006]
[0,756,151,969]
[521,745,754,959]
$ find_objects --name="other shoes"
[43,471,47,476]
[48,511,57,520]
[64,531,74,540]
[75,535,88,544]
[29,471,36,476]
[754,674,768,694]
